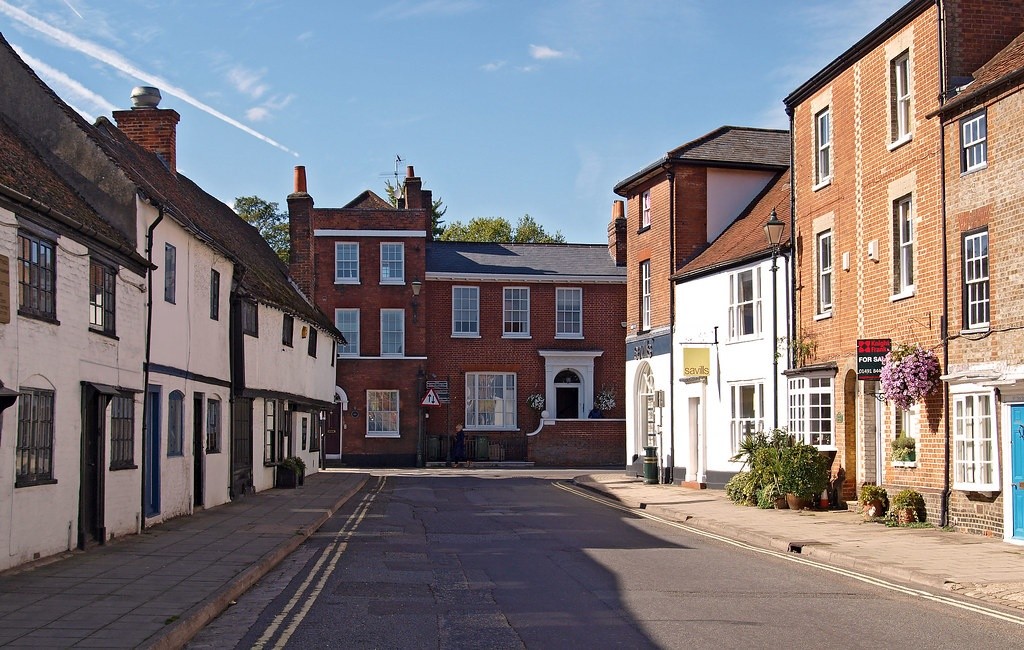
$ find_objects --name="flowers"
[526,393,545,411]
[593,385,619,411]
[879,341,943,412]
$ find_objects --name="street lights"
[414,370,425,467]
[761,204,786,435]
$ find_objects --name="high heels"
[451,464,459,468]
[464,461,472,468]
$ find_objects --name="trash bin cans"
[472,435,489,461]
[644,446,660,484]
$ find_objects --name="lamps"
[410,275,422,321]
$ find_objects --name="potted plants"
[857,485,887,517]
[891,490,923,526]
[280,456,306,488]
[728,430,831,509]
